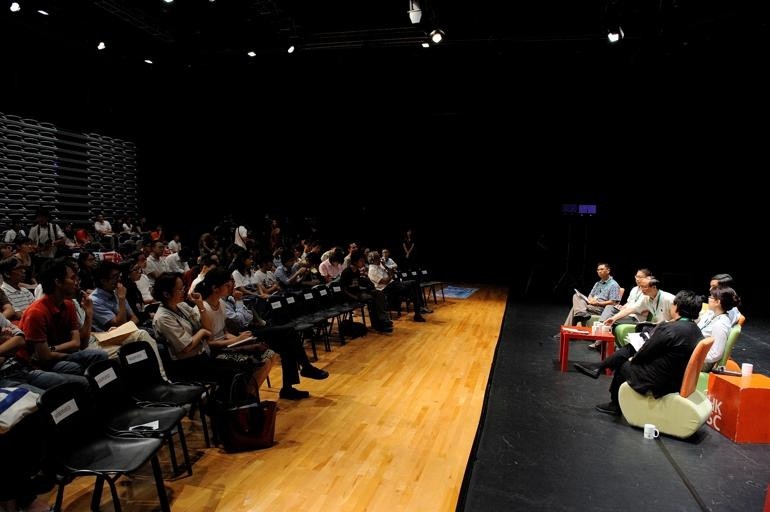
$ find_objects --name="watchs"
[50,346,56,352]
[199,308,206,313]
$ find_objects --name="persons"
[33,257,171,385]
[696,287,742,372]
[15,260,110,373]
[192,266,278,402]
[186,219,345,299]
[119,261,160,327]
[573,290,705,416]
[0,255,36,318]
[218,277,330,401]
[86,260,155,337]
[0,312,69,402]
[587,269,652,351]
[552,263,620,338]
[696,274,746,327]
[0,202,194,276]
[344,228,435,334]
[0,287,15,320]
[150,272,246,419]
[603,276,678,323]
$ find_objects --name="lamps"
[602,19,629,44]
[421,28,447,49]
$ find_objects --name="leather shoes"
[279,387,309,400]
[300,367,329,379]
[413,315,426,322]
[594,402,622,417]
[572,361,601,379]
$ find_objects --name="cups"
[594,323,604,331]
[644,424,659,439]
[592,326,602,335]
[602,326,612,333]
[742,363,753,376]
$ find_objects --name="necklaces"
[211,302,219,308]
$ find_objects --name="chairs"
[36,382,170,511]
[116,340,211,478]
[268,282,367,361]
[582,288,625,326]
[618,336,715,439]
[697,313,745,393]
[614,312,653,348]
[84,358,193,476]
[393,268,448,313]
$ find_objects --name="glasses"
[635,275,648,279]
[107,271,123,281]
[596,267,605,272]
[174,285,186,295]
[707,295,716,300]
[86,257,96,262]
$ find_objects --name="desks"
[560,325,615,376]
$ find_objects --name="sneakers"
[553,332,561,338]
[420,306,433,314]
[572,313,591,322]
[586,342,602,350]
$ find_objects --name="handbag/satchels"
[339,320,369,337]
[214,372,277,454]
